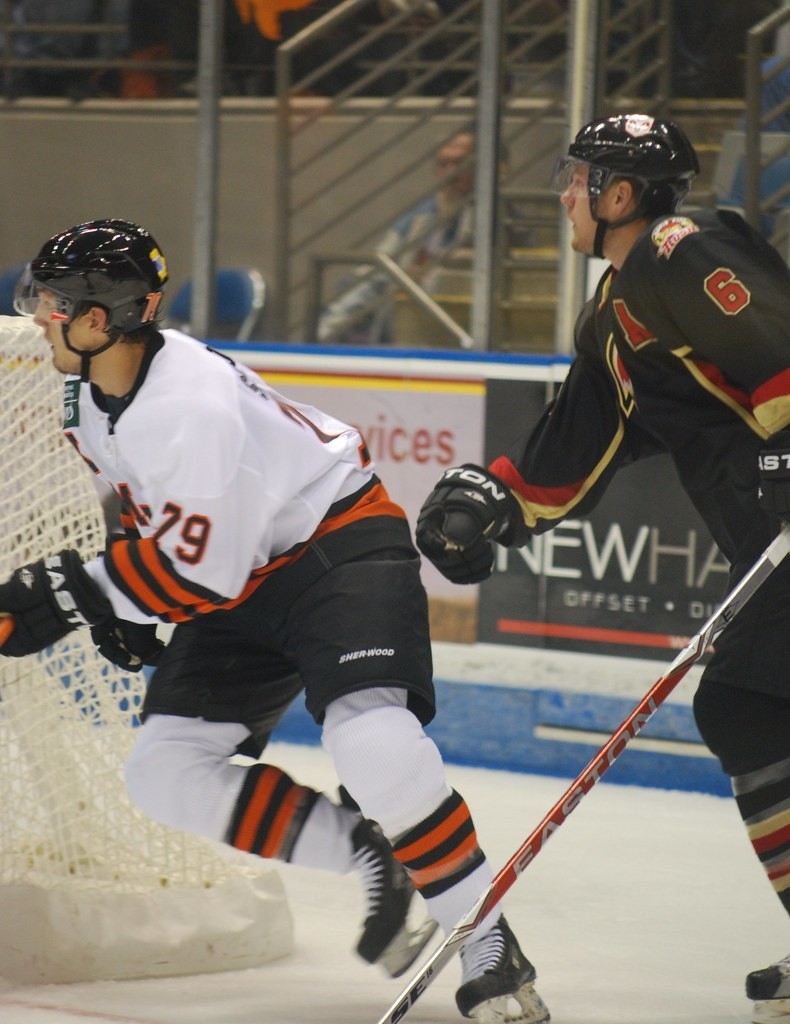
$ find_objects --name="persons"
[417,113,790,1023]
[0,0,789,98]
[0,219,555,1023]
[319,131,535,347]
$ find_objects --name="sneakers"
[339,784,442,979]
[745,955,789,1024]
[454,912,550,1024]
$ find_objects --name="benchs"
[0,0,790,357]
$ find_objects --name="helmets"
[30,219,169,332]
[566,112,698,198]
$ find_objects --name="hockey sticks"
[374,528,790,1024]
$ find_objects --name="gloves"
[0,549,102,657]
[756,448,790,536]
[413,463,532,585]
[92,618,166,671]
